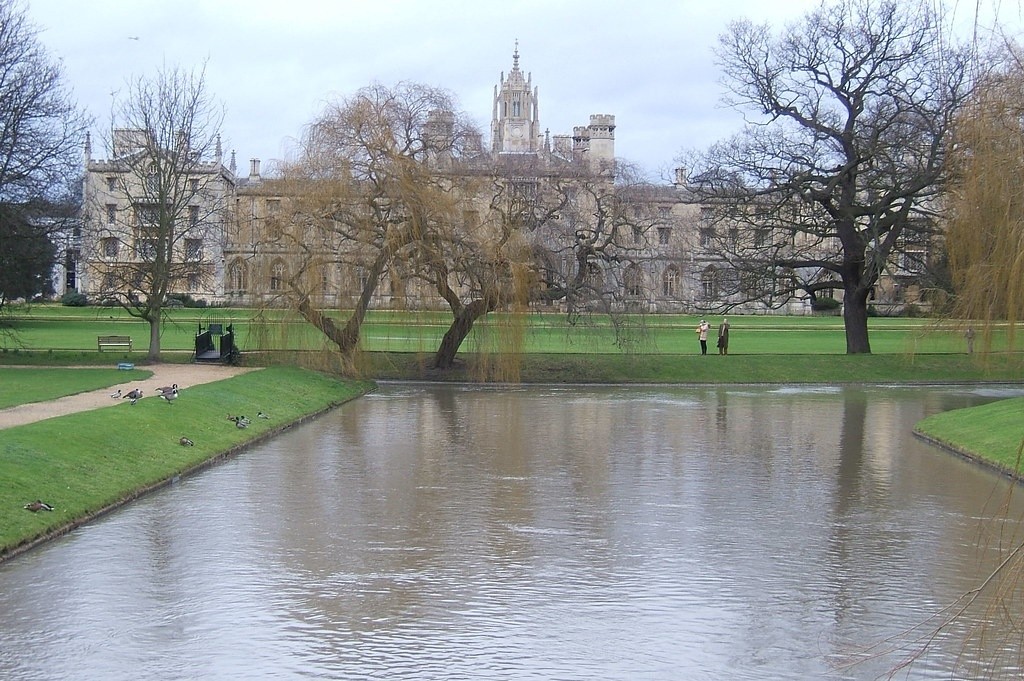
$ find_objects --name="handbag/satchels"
[696,328,700,333]
[717,337,724,347]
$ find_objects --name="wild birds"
[226,412,251,429]
[123,388,143,405]
[154,384,178,404]
[257,412,269,419]
[23,500,55,512]
[180,437,194,447]
[109,390,122,400]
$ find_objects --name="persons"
[717,318,731,356]
[695,320,710,356]
[964,326,976,353]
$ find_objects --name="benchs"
[98,336,133,352]
